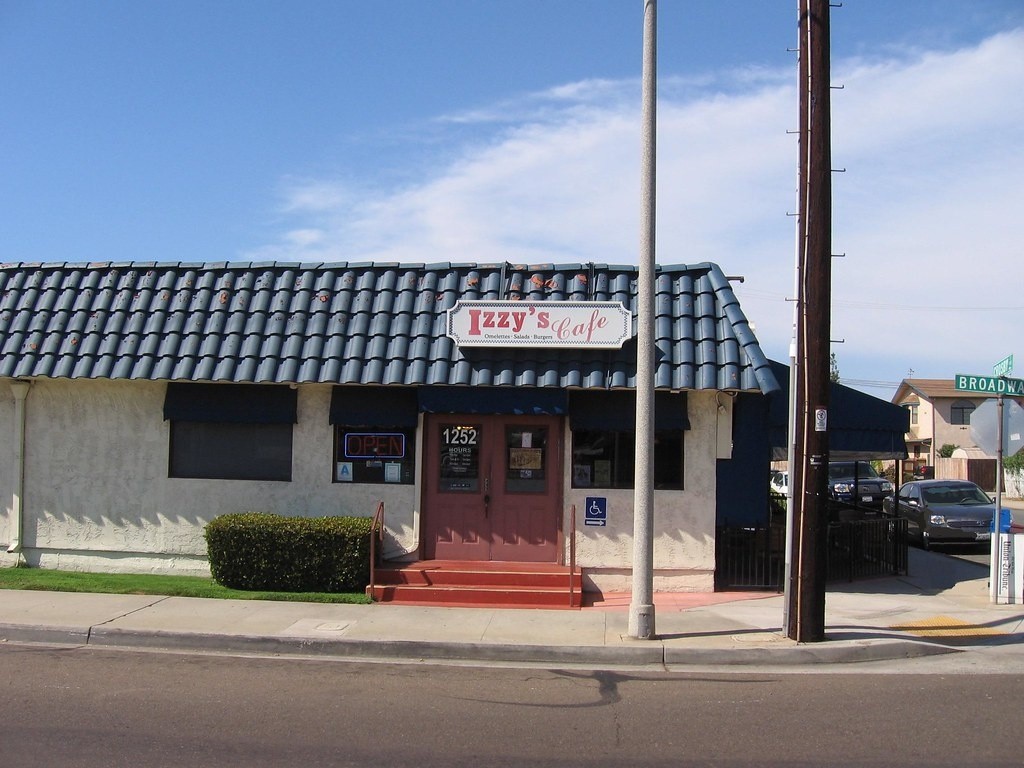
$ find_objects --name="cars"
[770,472,788,493]
[883,480,997,552]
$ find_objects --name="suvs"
[828,462,894,505]
